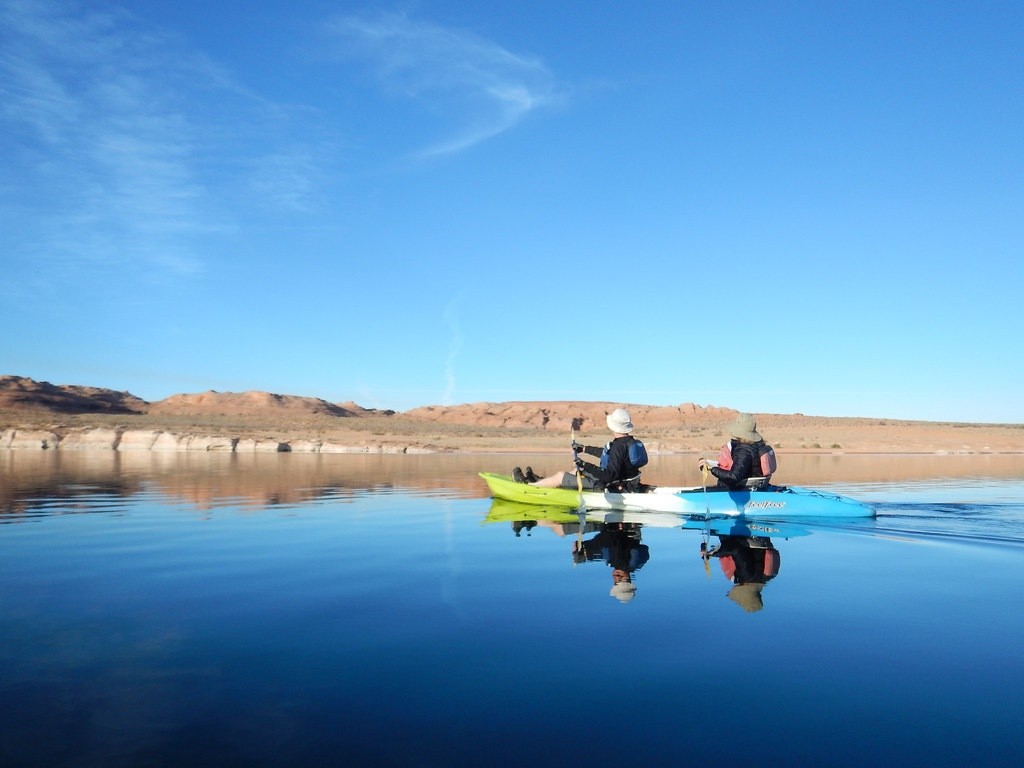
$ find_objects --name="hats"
[609,581,637,601]
[727,583,763,613]
[726,413,762,442]
[606,408,633,433]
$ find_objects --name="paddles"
[701,467,709,517]
[569,423,589,511]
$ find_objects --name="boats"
[481,494,813,539]
[478,470,877,519]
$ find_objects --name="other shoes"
[512,467,528,484]
[525,466,543,483]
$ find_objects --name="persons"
[572,523,642,602]
[700,535,774,611]
[698,414,771,489]
[511,408,641,491]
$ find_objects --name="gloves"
[572,443,584,453]
[572,551,586,563]
[573,457,586,468]
